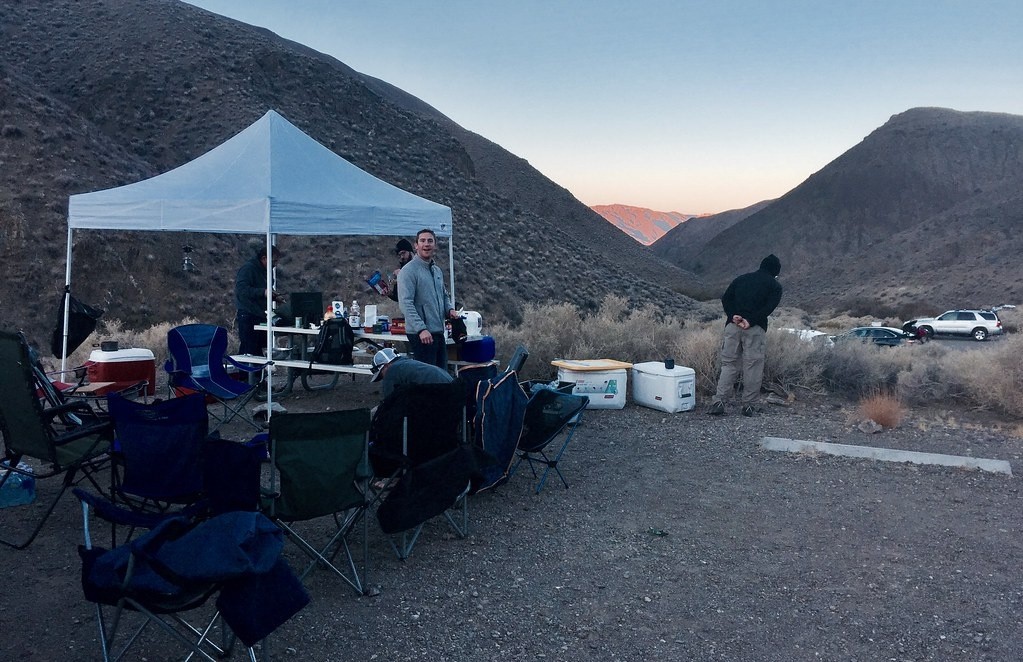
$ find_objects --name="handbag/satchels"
[51,289,103,359]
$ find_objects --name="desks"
[254,324,483,393]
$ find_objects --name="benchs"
[230,355,375,375]
[277,347,407,357]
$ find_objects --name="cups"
[664,357,675,369]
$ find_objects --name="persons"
[915,326,930,344]
[235,245,287,392]
[366,347,455,491]
[706,253,783,418]
[396,229,461,372]
[380,238,415,302]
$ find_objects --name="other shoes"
[707,402,724,414]
[260,381,275,392]
[743,407,753,416]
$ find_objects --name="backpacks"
[310,317,354,365]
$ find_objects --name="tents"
[59,109,455,422]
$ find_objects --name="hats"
[761,253,781,278]
[370,348,400,383]
[396,239,413,255]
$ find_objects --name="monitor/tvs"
[290,292,324,330]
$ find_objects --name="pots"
[91,342,119,351]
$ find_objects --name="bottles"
[324,306,336,321]
[0,461,36,508]
[349,301,361,329]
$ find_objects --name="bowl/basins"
[262,347,293,360]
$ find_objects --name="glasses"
[774,275,778,279]
[398,251,407,257]
[371,363,384,373]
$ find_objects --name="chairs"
[0,324,590,662]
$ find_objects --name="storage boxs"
[632,361,695,412]
[558,366,627,409]
[88,348,155,396]
[175,364,239,405]
